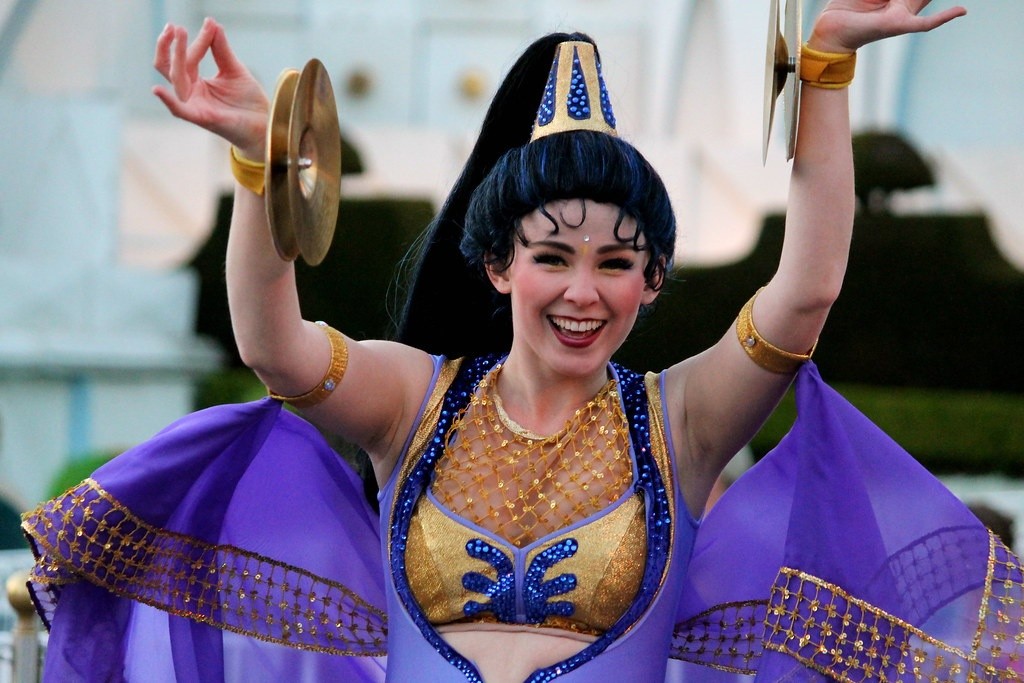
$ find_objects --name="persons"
[21,0,1024,683]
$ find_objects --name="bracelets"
[229,142,267,197]
[798,40,857,90]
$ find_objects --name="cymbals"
[757,0,805,169]
[262,56,345,267]
